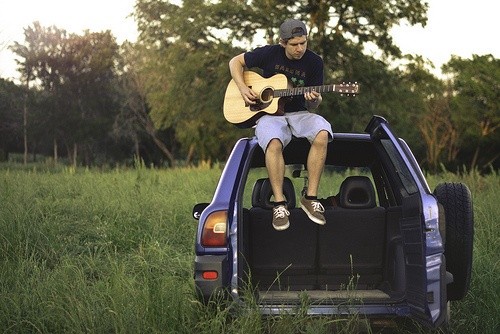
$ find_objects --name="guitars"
[222,70,360,129]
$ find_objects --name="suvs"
[192,115,474,330]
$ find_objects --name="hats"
[279,20,306,38]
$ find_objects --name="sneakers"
[299,194,326,225]
[272,202,291,230]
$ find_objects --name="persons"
[229,20,334,230]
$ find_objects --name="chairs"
[244,176,385,292]
